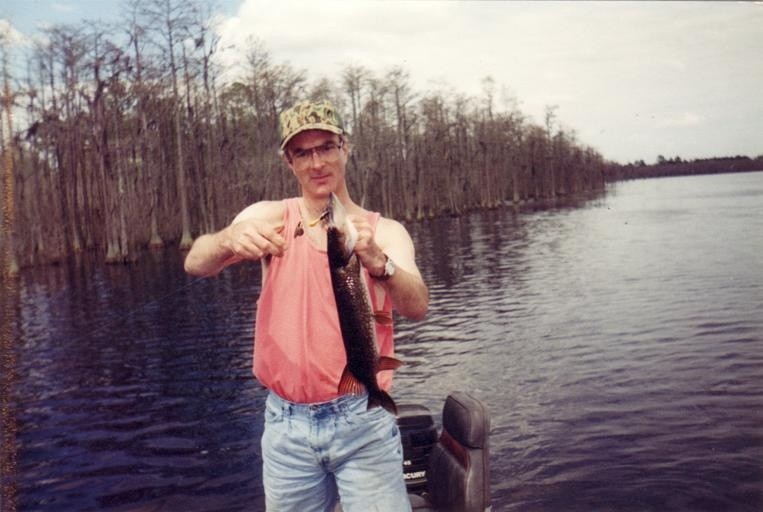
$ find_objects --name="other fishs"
[325,191,403,417]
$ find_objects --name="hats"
[278,101,343,149]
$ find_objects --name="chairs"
[335,392,490,511]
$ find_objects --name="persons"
[180,101,436,512]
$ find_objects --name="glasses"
[289,141,344,162]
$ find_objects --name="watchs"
[368,252,395,279]
